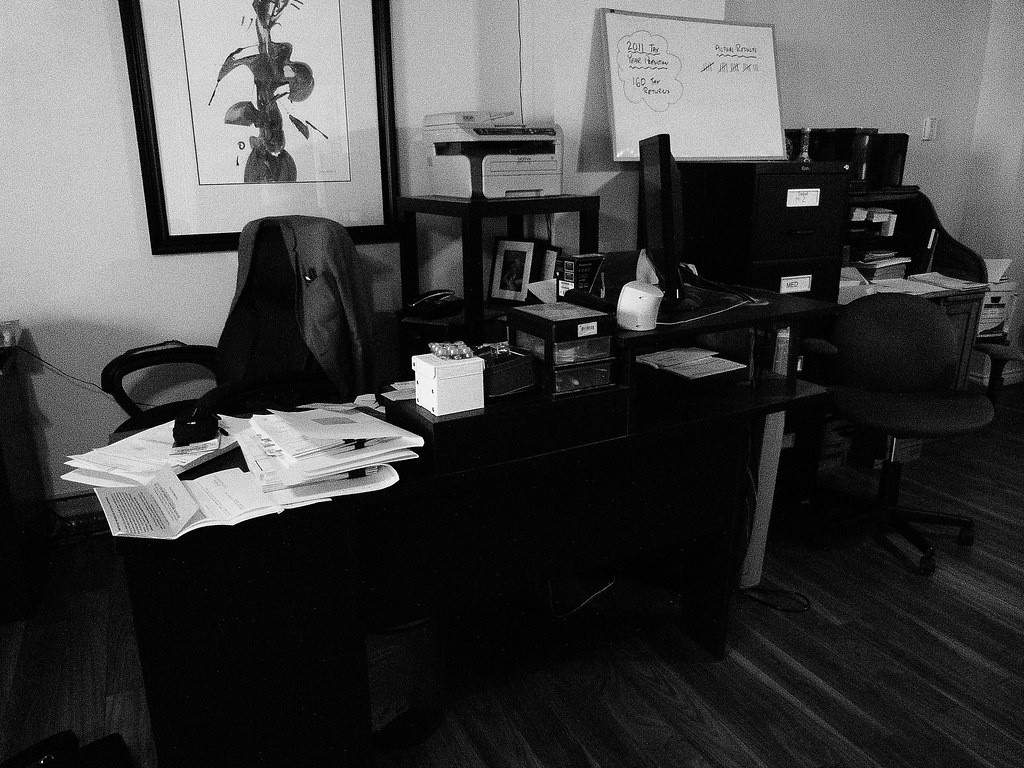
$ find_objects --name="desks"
[0,345,47,621]
[108,280,836,768]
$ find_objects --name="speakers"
[616,282,664,331]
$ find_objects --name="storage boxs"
[978,281,1020,339]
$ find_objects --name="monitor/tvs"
[752,161,844,268]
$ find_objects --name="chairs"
[812,291,994,579]
[99,216,325,447]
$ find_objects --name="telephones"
[407,289,464,321]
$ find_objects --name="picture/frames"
[118,0,403,256]
[488,236,537,306]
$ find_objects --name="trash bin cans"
[0,346,49,543]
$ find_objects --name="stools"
[973,341,1024,406]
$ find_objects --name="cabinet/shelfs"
[400,194,600,378]
[788,125,988,463]
[637,134,847,305]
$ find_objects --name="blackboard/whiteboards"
[600,7,787,162]
